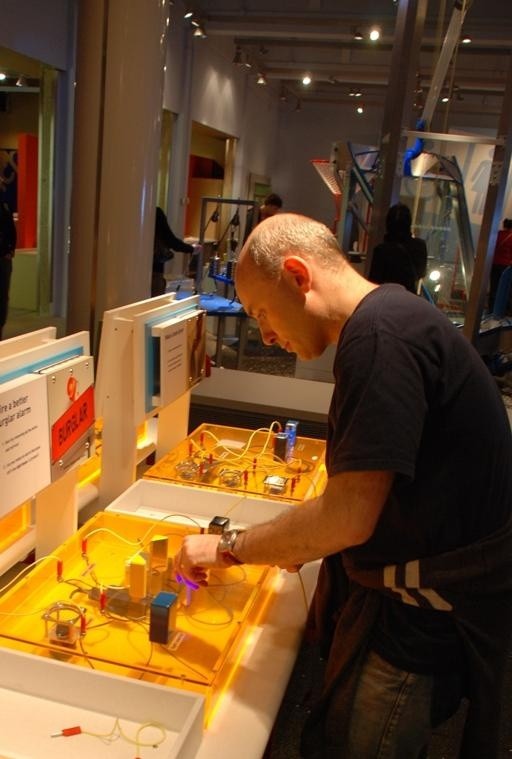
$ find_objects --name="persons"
[243,194,282,245]
[174,212,512,759]
[0,195,17,334]
[152,207,203,297]
[367,203,427,295]
[488,218,511,315]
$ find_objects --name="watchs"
[217,529,246,565]
